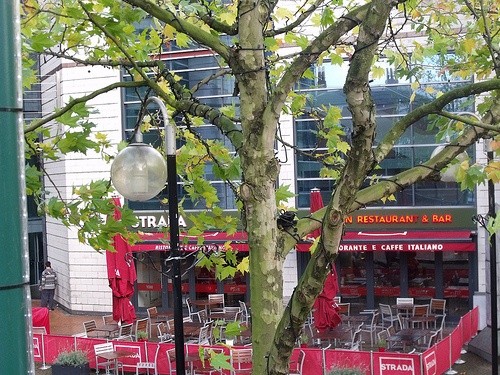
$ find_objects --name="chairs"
[33,293,446,375]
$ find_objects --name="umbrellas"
[105,192,136,328]
[310,187,343,337]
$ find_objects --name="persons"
[39,261,57,311]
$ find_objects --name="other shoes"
[49,309,54,311]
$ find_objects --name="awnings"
[125,230,476,252]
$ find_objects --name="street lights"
[430,112,499,375]
[110,95,187,375]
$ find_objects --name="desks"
[166,326,200,338]
[411,317,438,329]
[91,325,117,338]
[183,322,204,327]
[394,329,431,345]
[391,304,414,317]
[385,336,422,351]
[192,300,222,312]
[205,312,235,322]
[341,315,370,327]
